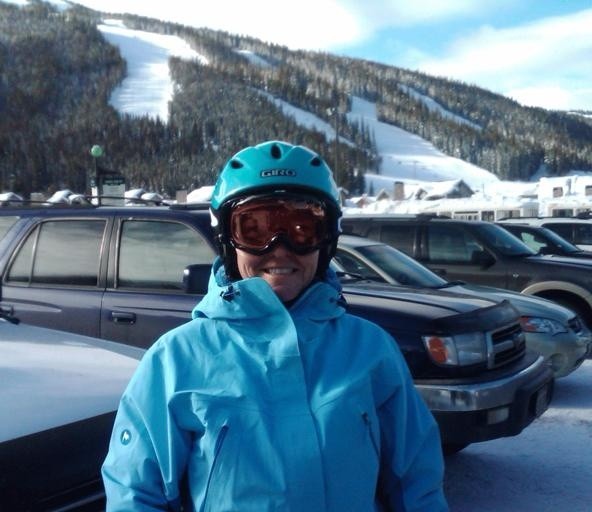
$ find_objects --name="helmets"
[209,139,343,218]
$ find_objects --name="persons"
[101,139,451,512]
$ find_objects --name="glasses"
[220,191,332,256]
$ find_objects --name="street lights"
[90,145,103,206]
